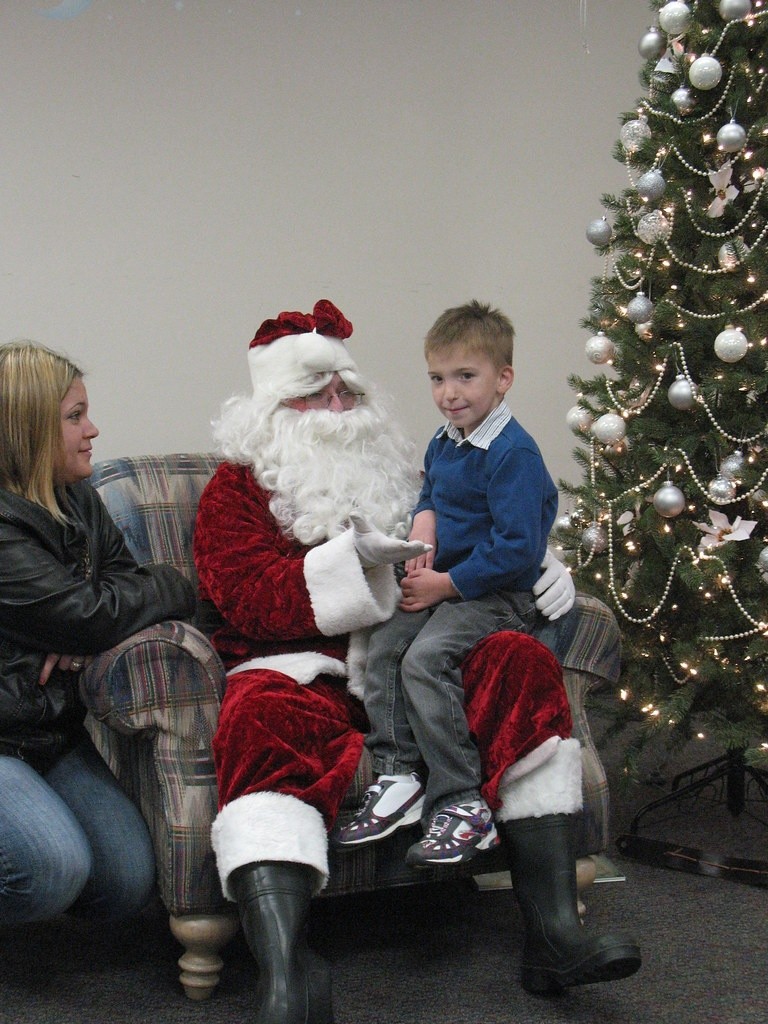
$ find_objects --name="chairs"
[72,454,620,996]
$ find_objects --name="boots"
[498,813,643,992]
[234,860,334,1024]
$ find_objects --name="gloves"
[351,511,433,571]
[534,550,574,623]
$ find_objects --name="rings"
[72,660,82,668]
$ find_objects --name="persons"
[0,340,197,925]
[193,300,642,1024]
[337,300,558,871]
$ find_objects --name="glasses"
[290,390,365,411]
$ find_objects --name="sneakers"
[406,797,499,866]
[337,778,426,845]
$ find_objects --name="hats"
[246,299,356,398]
